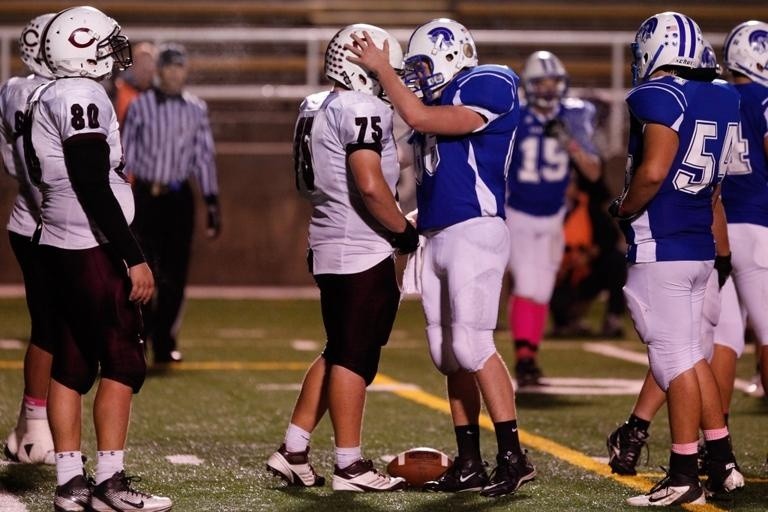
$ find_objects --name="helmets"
[523,50,569,104]
[19,12,79,79]
[43,3,132,80]
[721,19,767,85]
[326,22,404,97]
[405,17,478,99]
[633,8,716,84]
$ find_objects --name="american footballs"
[387,447,453,489]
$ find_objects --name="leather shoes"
[154,340,185,364]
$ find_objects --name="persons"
[609,12,767,512]
[116,44,225,366]
[23,7,175,511]
[266,18,539,496]
[505,49,627,389]
[2,13,91,465]
[112,51,159,128]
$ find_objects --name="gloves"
[205,210,224,239]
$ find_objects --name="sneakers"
[264,444,325,488]
[332,459,406,492]
[481,453,537,497]
[514,357,546,388]
[690,460,744,492]
[605,418,648,475]
[626,475,706,507]
[424,456,486,491]
[2,429,88,466]
[53,472,172,512]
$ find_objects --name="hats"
[156,43,190,67]
